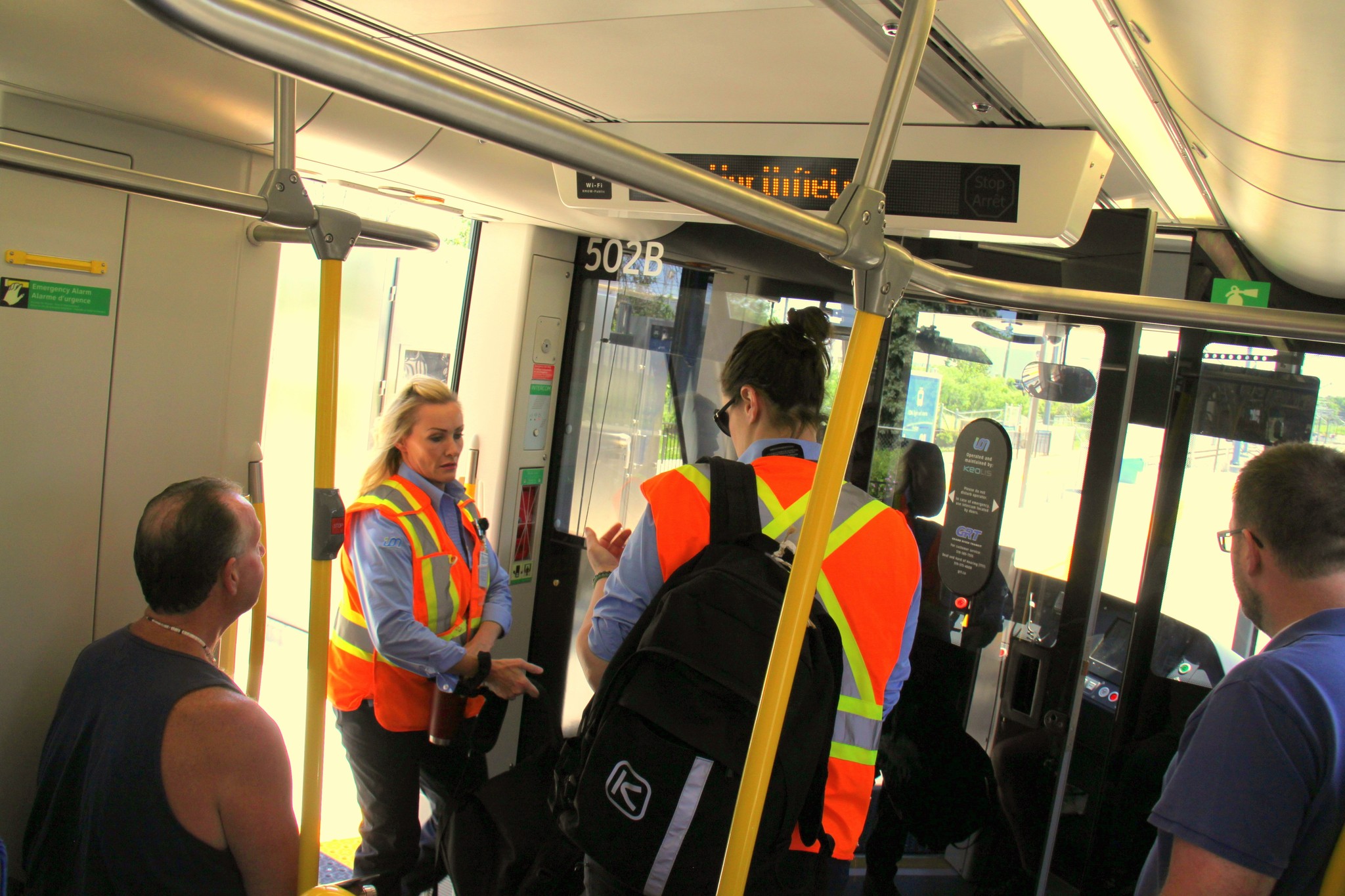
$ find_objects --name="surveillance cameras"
[1045,324,1065,344]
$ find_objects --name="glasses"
[713,394,736,437]
[1217,528,1265,553]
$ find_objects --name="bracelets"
[591,570,611,588]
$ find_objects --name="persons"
[575,307,923,896]
[328,374,542,896]
[1134,443,1345,896]
[18,476,300,896]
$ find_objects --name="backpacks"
[570,458,845,895]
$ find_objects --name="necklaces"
[144,616,216,661]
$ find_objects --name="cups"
[428,683,460,747]
